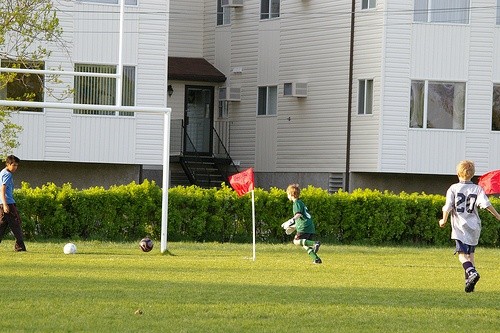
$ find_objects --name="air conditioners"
[220,0,244,9]
[283,81,308,97]
[218,86,241,103]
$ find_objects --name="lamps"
[167,85,173,98]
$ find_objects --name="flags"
[228,168,254,196]
[478,170,500,194]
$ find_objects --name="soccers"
[64,243,77,254]
[139,237,153,252]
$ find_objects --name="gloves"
[286,224,296,235]
[281,219,295,229]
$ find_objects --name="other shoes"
[465,271,480,293]
[311,258,322,264]
[314,241,320,254]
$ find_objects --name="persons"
[438,161,500,292]
[0,155,27,251]
[281,184,322,264]
